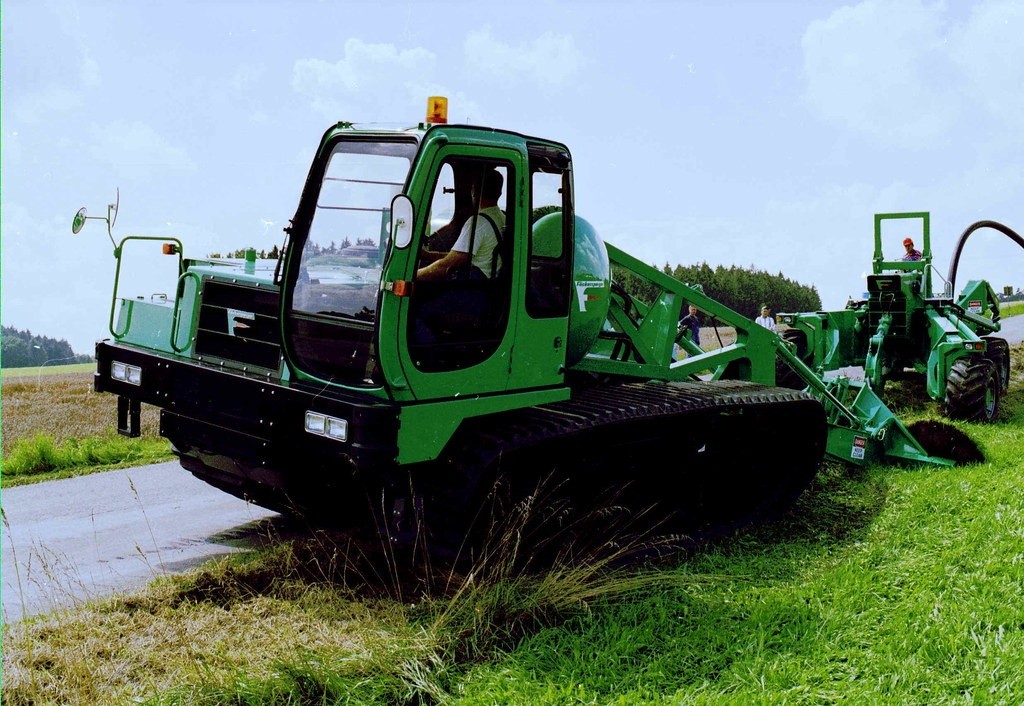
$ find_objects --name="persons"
[755,307,775,335]
[901,237,922,262]
[412,170,507,377]
[678,304,701,359]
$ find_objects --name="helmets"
[903,238,913,246]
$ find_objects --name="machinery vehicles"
[72,97,960,581]
[776,211,1024,425]
